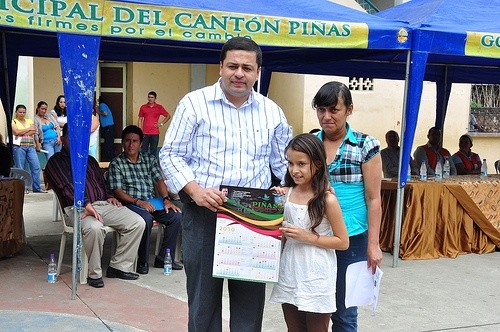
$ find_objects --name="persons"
[268,132,350,332]
[89,105,100,160]
[380,130,418,178]
[50,94,68,136]
[12,104,48,194]
[452,133,483,175]
[413,126,458,176]
[95,96,115,161]
[34,101,61,160]
[309,81,383,332]
[137,91,171,155]
[159,37,295,332]
[45,123,146,287]
[108,125,183,274]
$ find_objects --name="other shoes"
[33,189,48,193]
[24,189,29,194]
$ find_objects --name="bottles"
[481,159,487,177]
[47,254,57,283]
[435,160,442,181]
[164,249,172,276]
[420,160,427,180]
[443,158,450,178]
[407,162,411,181]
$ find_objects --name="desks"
[381,173,500,260]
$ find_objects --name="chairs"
[51,190,122,284]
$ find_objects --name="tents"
[0,1,410,298]
[253,0,500,268]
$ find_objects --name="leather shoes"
[88,276,104,288]
[154,256,183,270]
[106,267,139,280]
[137,261,149,274]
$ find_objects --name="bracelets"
[134,198,140,204]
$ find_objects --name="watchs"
[163,196,171,201]
[161,123,163,126]
